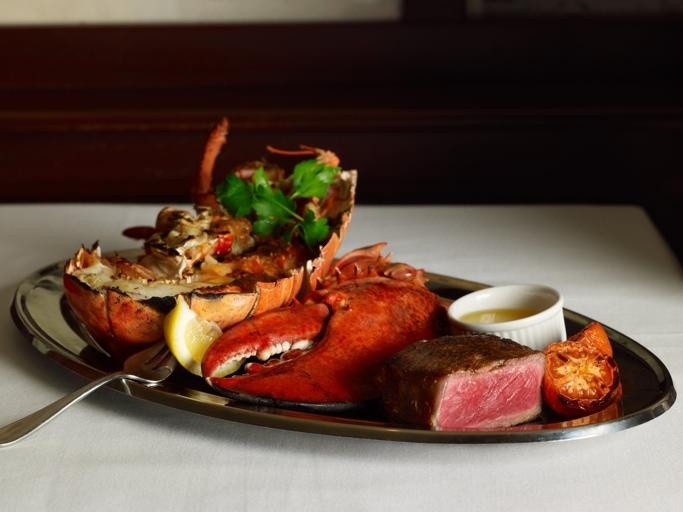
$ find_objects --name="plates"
[11,249,677,444]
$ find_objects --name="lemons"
[162,293,246,378]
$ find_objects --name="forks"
[0,341,177,446]
